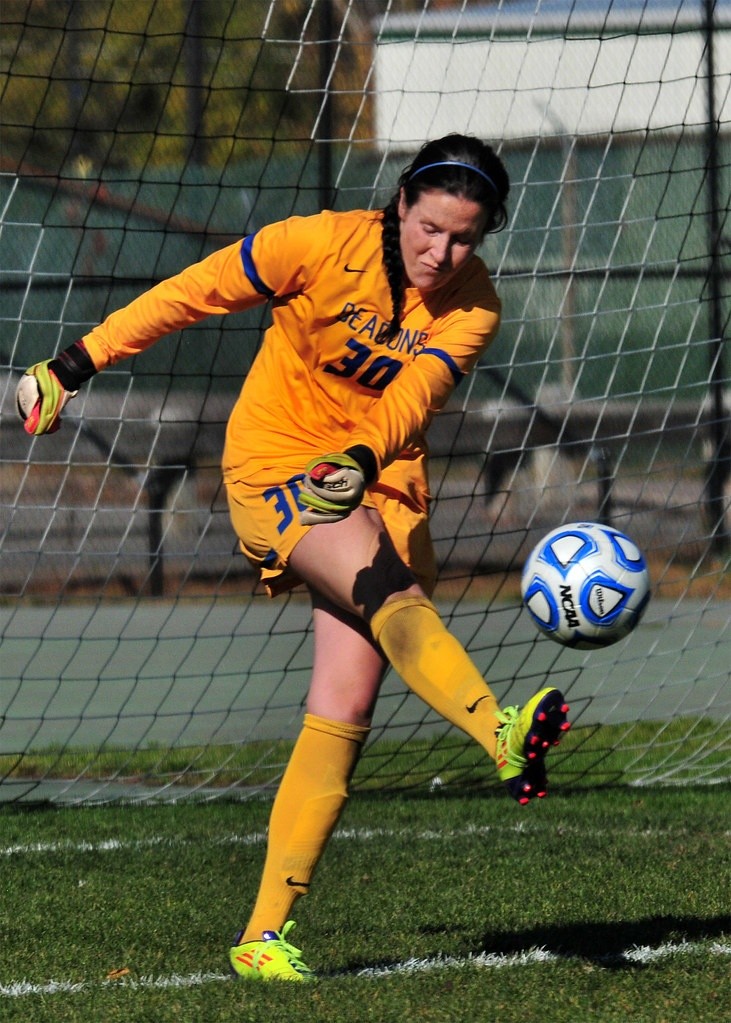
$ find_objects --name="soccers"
[519,522,651,650]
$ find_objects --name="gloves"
[22,342,98,436]
[299,444,379,525]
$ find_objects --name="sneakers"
[227,927,319,986]
[495,688,569,804]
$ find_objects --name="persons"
[13,131,573,984]
